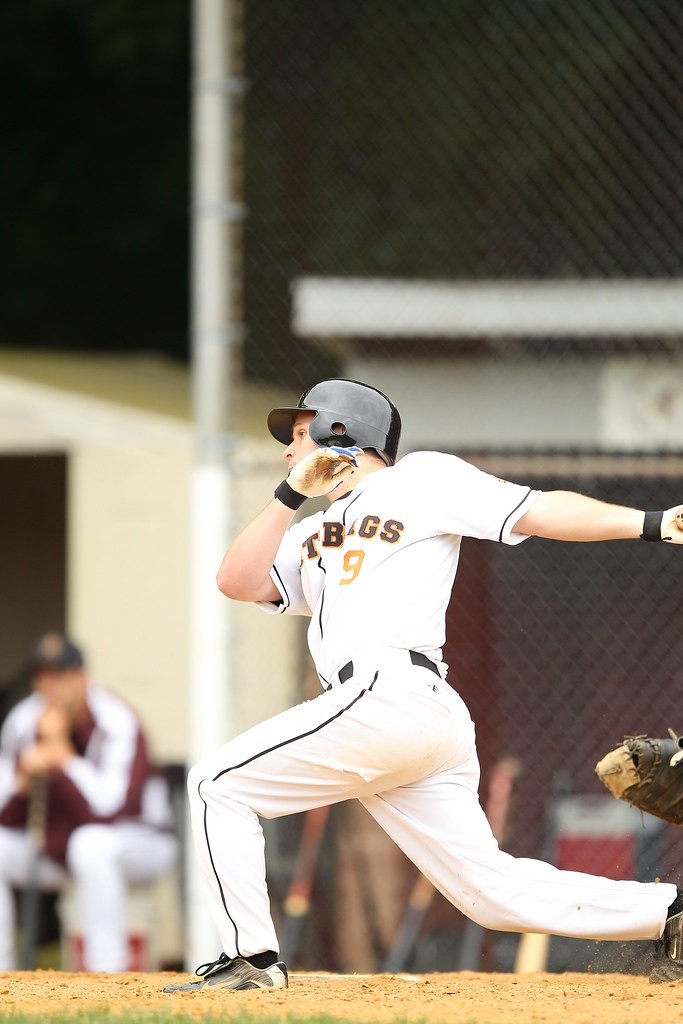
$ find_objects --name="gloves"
[275,446,364,510]
[640,505,683,544]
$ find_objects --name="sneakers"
[163,952,288,993]
[649,888,683,984]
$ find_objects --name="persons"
[158,382,683,996]
[0,628,178,975]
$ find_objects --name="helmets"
[267,379,401,467]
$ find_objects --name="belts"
[325,650,441,692]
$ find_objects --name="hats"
[25,633,81,670]
